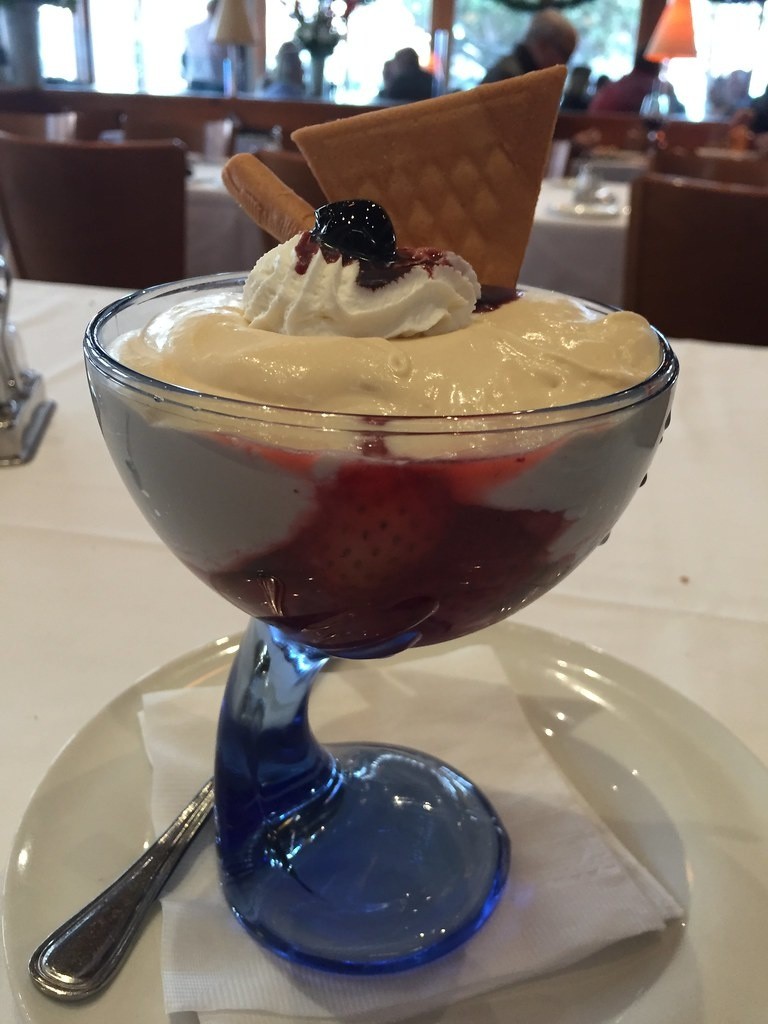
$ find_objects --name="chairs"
[2,130,185,291]
[619,172,768,346]
[3,103,77,148]
[170,113,241,157]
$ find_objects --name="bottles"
[572,160,599,205]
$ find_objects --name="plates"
[549,198,623,220]
[6,623,768,1024]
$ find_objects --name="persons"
[181,0,767,137]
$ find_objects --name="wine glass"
[73,262,681,977]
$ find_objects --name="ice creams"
[104,64,667,661]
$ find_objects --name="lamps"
[207,0,260,102]
[641,0,700,118]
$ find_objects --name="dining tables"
[187,147,264,279]
[514,172,634,307]
[1,274,766,1023]
